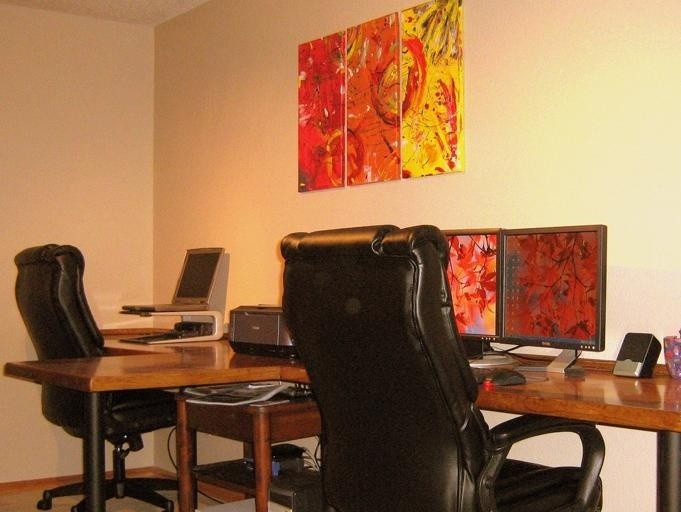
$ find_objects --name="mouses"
[490,370,526,386]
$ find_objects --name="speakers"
[613,333,662,378]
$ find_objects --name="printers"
[228,305,301,360]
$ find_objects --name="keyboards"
[471,367,496,384]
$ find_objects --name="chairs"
[14,243,180,512]
[280,224,606,509]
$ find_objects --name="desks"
[174,396,322,511]
[4,333,679,512]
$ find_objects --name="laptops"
[122,248,226,312]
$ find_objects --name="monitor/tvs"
[502,225,607,375]
[441,228,521,368]
